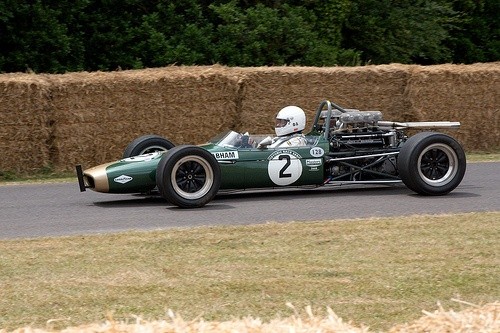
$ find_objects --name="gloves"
[241,135,258,149]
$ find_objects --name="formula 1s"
[75,99,467,209]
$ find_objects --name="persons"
[239,106,307,149]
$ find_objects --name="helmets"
[275,106,306,137]
